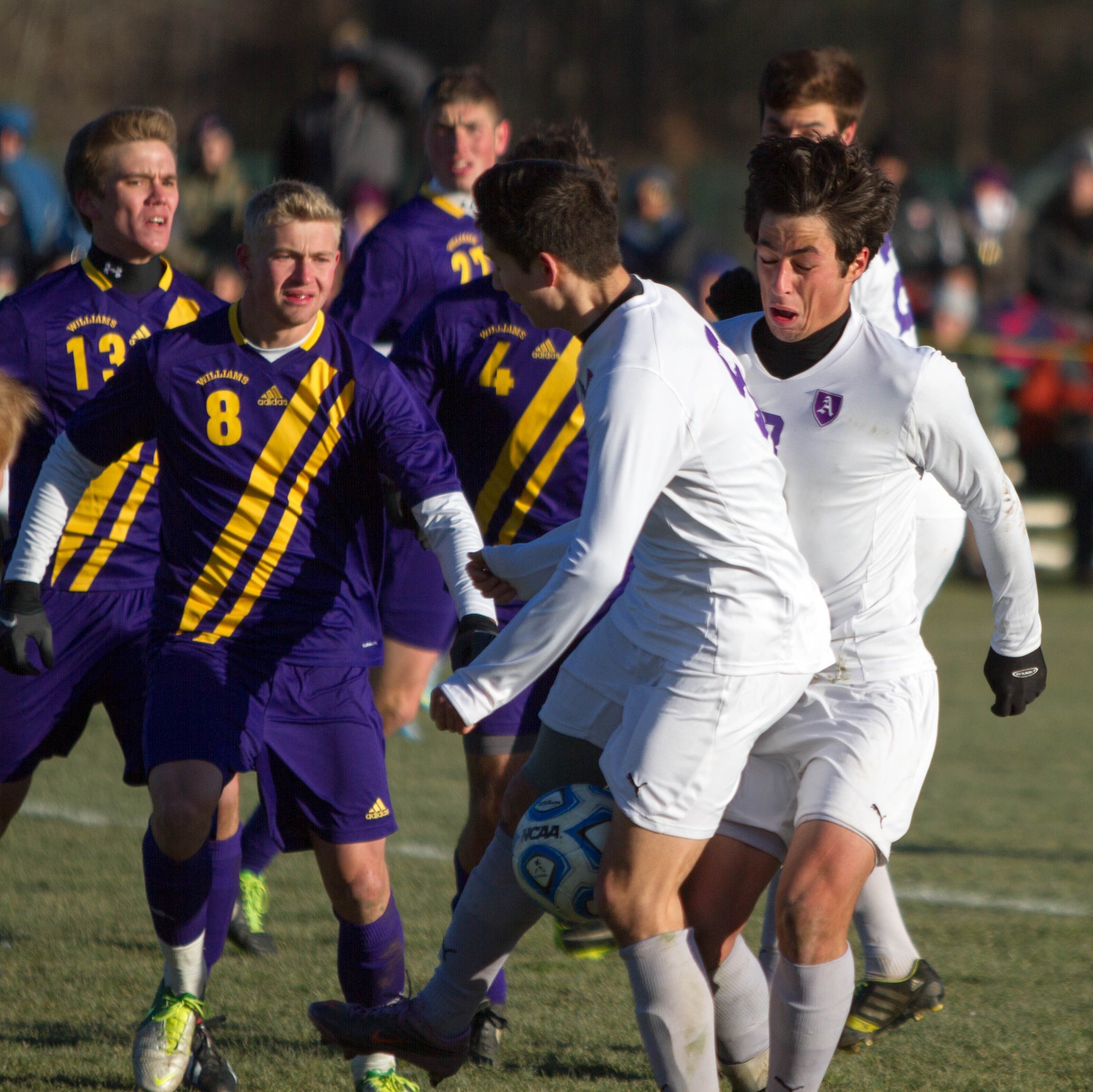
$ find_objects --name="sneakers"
[181,1018,237,1091]
[837,958,946,1052]
[307,997,471,1086]
[553,918,619,963]
[135,977,205,1092]
[462,1003,508,1067]
[350,1070,420,1092]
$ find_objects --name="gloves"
[450,615,500,673]
[0,579,55,677]
[978,647,1045,715]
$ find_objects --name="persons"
[367,118,627,1070]
[2,22,1093,594]
[467,134,1053,1092]
[301,124,844,1089]
[0,182,515,1090]
[217,78,651,966]
[0,106,244,1092]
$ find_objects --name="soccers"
[510,781,611,928]
